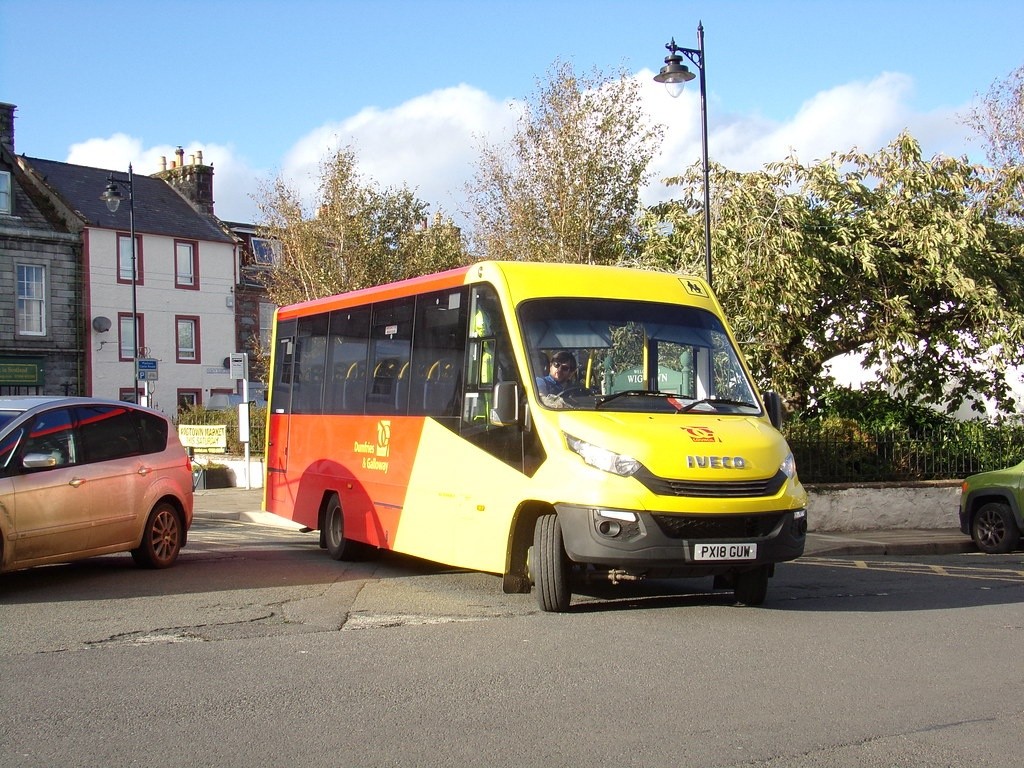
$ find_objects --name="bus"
[262,261,806,612]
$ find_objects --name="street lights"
[653,18,714,288]
[100,161,139,406]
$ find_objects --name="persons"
[535,351,597,409]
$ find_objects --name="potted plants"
[192,458,229,490]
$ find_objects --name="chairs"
[395,359,432,411]
[319,362,348,410]
[298,365,323,408]
[368,358,402,411]
[423,358,461,411]
[515,351,550,416]
[342,361,377,410]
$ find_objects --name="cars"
[0,395,194,579]
[958,459,1024,555]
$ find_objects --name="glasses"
[552,362,570,371]
